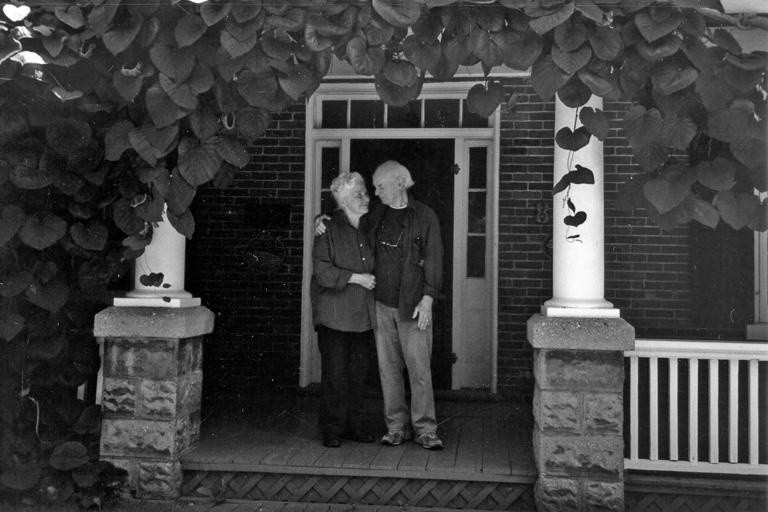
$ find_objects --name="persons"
[310,171,377,446]
[312,159,445,451]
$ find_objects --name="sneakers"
[379,428,413,446]
[413,432,444,450]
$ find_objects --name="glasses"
[378,231,403,248]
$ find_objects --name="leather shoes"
[323,426,341,447]
[344,424,375,443]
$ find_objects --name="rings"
[426,319,429,322]
